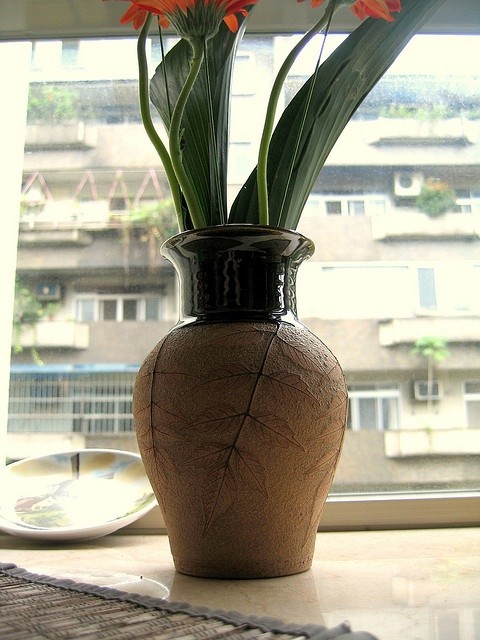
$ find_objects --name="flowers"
[118,0,447,235]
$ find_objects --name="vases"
[132,223,349,581]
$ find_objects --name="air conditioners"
[33,272,62,303]
[390,167,423,199]
[413,378,443,404]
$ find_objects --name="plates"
[1,449,160,541]
